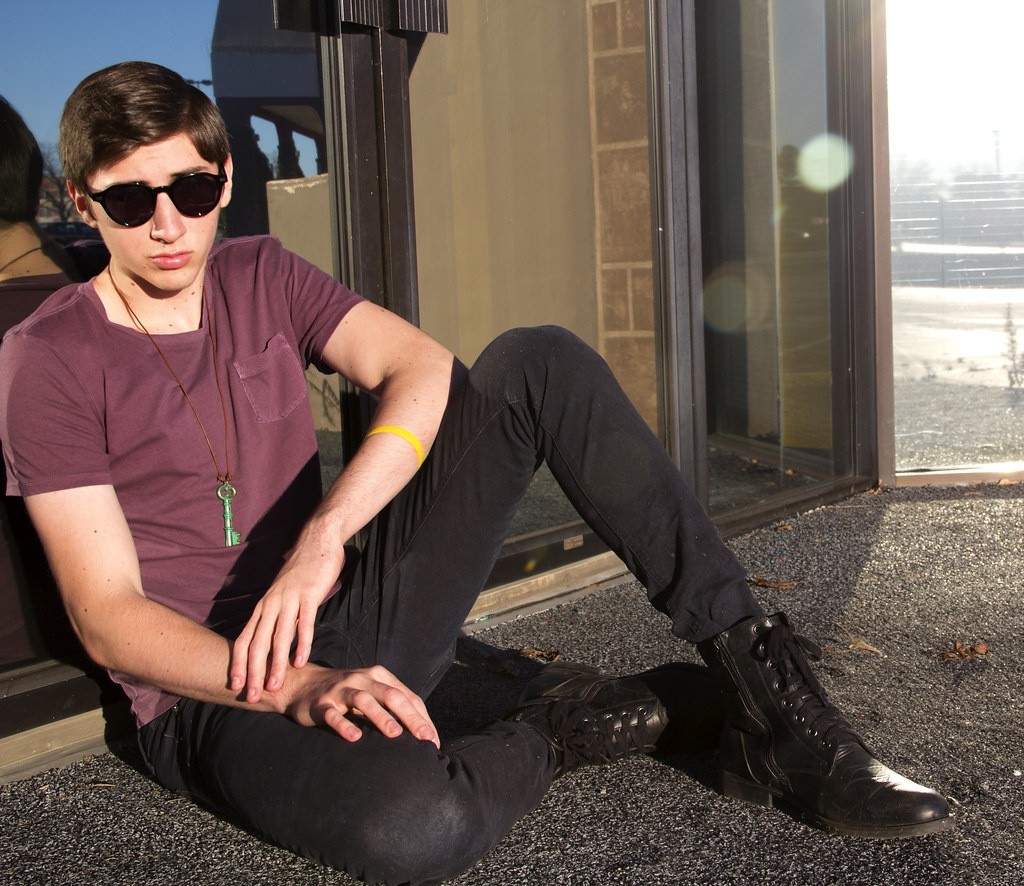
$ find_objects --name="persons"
[0,94,112,350]
[0,62,957,886]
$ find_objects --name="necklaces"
[0,246,43,274]
[105,264,241,547]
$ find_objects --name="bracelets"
[363,425,425,470]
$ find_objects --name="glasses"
[79,163,228,227]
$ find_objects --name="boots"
[504,661,727,782]
[696,611,955,838]
